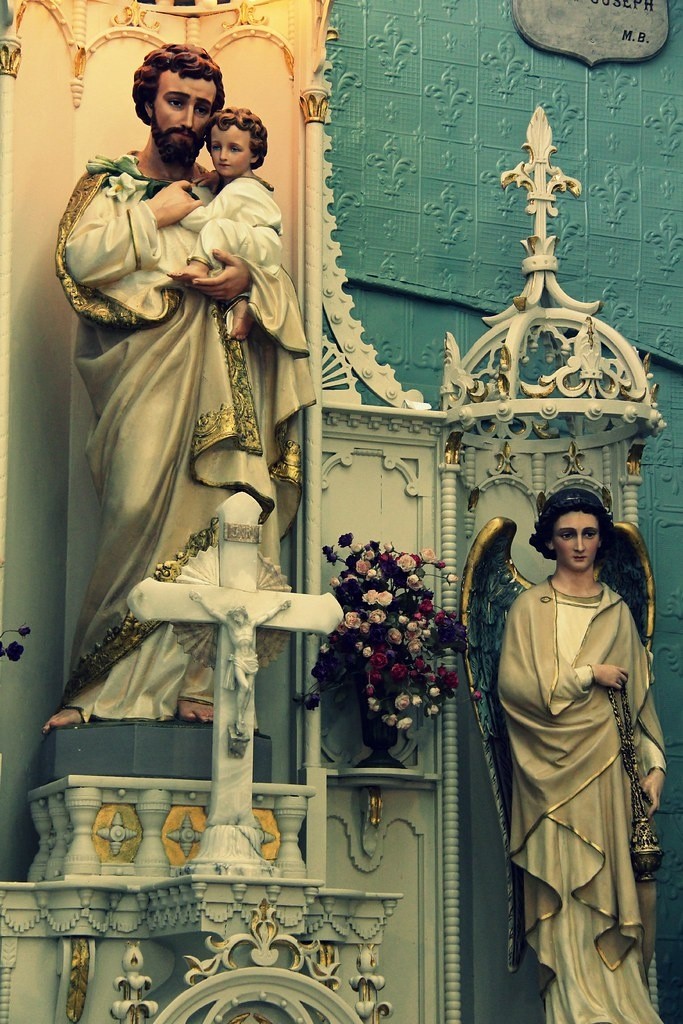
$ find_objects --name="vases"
[356,686,407,768]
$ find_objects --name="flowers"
[291,530,482,729]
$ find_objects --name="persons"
[32,43,323,732]
[491,488,674,1024]
[167,106,283,342]
